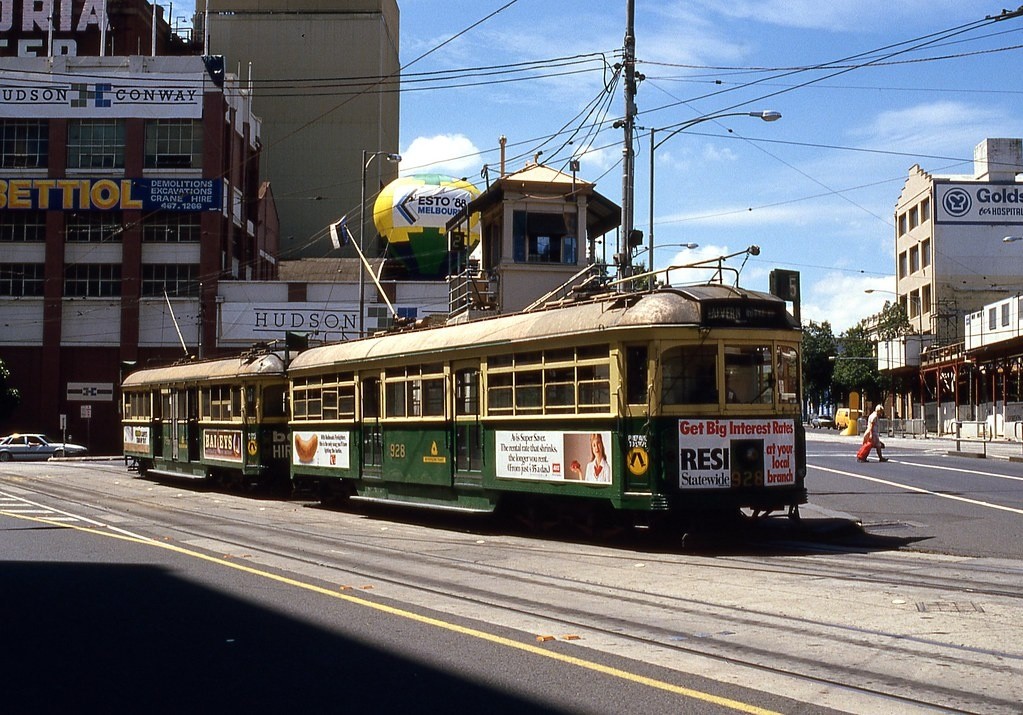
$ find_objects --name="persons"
[855,404,889,463]
[570,434,609,483]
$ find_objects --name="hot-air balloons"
[373,173,481,281]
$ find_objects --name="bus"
[121,247,808,543]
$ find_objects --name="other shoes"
[879,458,888,463]
[858,458,869,462]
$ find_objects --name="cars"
[811,415,834,430]
[0,434,89,462]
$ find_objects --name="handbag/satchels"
[858,439,873,460]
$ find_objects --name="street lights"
[864,289,927,438]
[360,149,403,338]
[650,110,782,294]
[629,244,698,292]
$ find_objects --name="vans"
[835,408,863,431]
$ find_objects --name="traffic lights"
[629,230,643,244]
